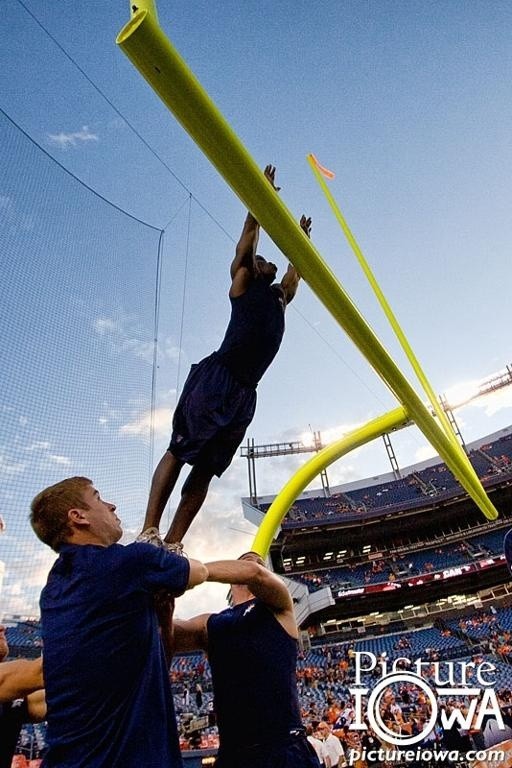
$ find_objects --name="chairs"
[0,431,512,749]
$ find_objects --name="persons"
[0,622,50,768]
[169,648,222,754]
[10,721,51,768]
[131,162,314,558]
[14,617,46,660]
[27,476,210,768]
[157,550,325,767]
[255,438,512,767]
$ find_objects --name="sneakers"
[133,526,163,548]
[160,539,188,560]
